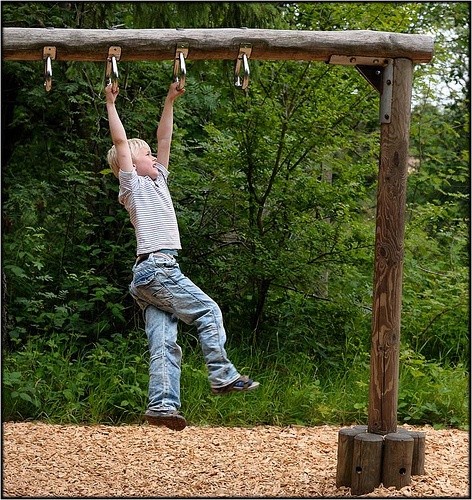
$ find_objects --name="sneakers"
[145,409,186,431]
[210,375,260,393]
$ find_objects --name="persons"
[104,81,260,432]
